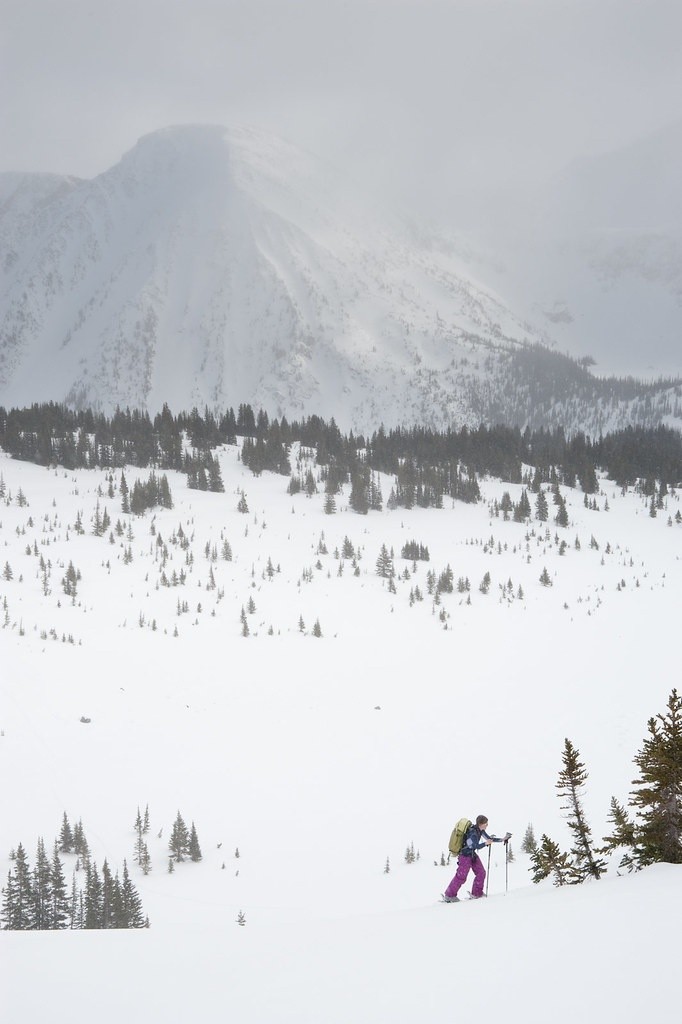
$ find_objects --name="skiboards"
[438,889,474,903]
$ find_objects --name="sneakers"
[472,894,487,899]
[444,895,460,902]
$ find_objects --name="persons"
[441,814,512,903]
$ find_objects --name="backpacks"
[447,818,479,853]
